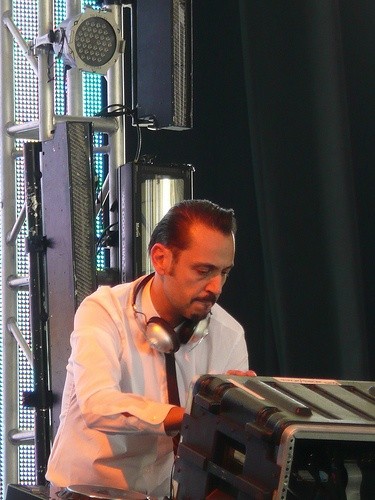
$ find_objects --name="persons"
[45,199,257,499]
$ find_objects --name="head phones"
[132,272,212,353]
